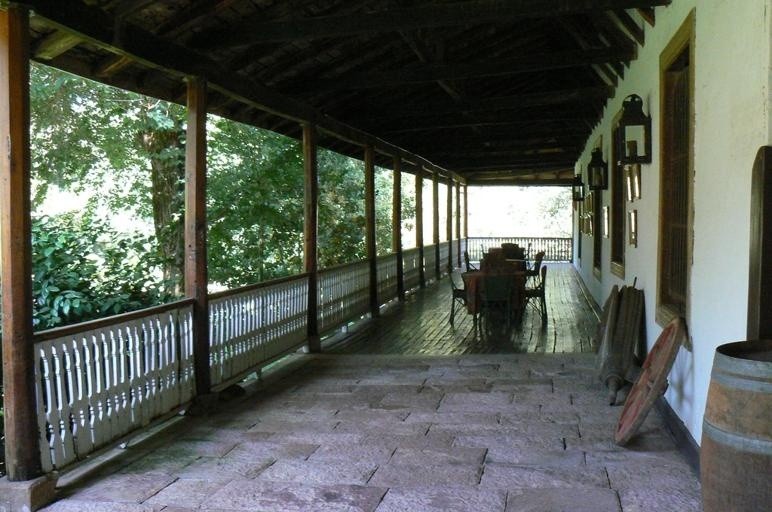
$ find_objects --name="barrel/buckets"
[700,338,772,512]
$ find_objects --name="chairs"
[448,243,547,331]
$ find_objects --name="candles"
[571,93,651,201]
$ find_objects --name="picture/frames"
[579,161,641,247]
